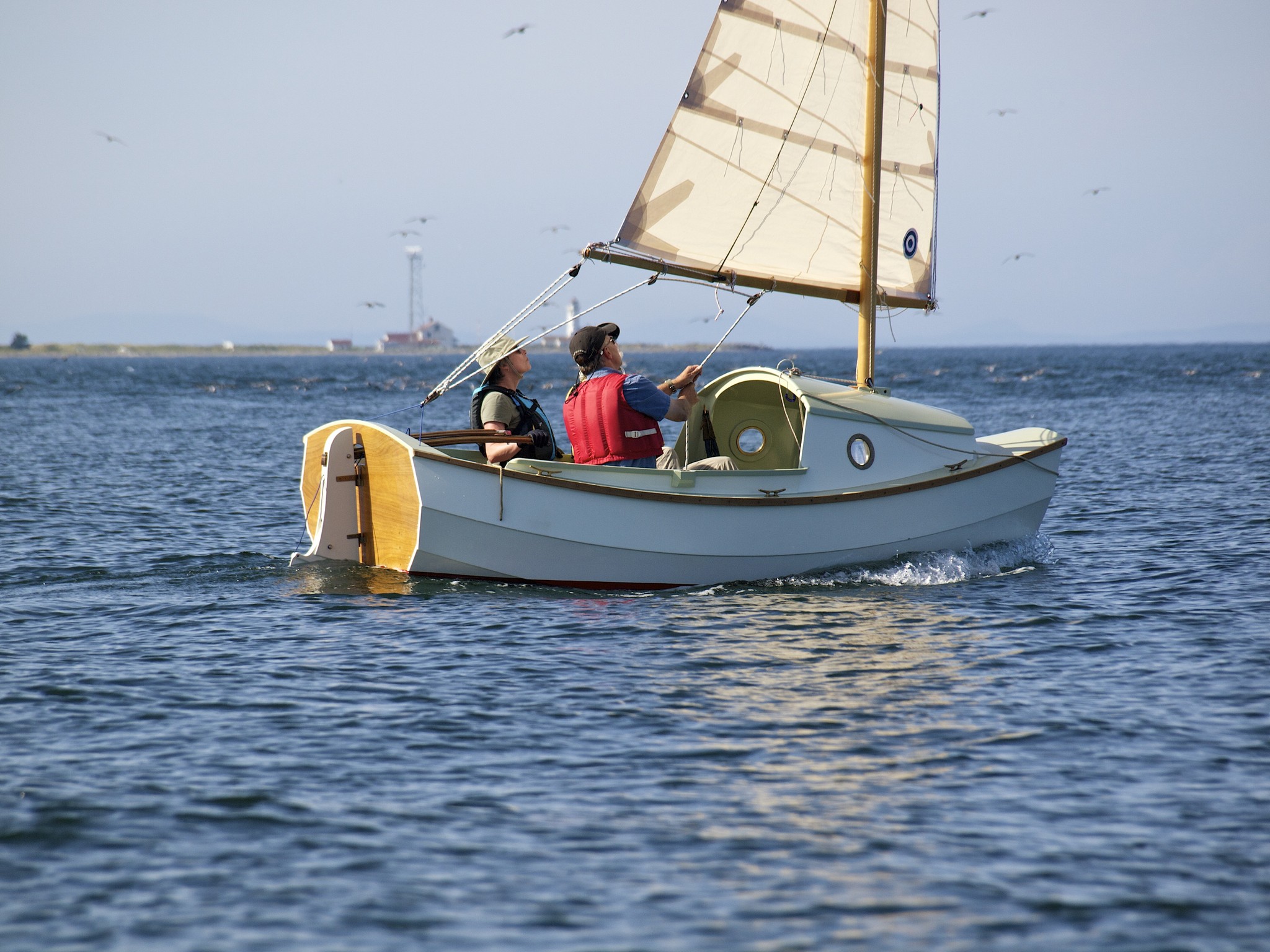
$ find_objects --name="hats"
[570,322,620,367]
[475,333,529,387]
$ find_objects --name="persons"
[563,323,739,471]
[469,332,565,468]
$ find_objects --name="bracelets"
[666,379,677,394]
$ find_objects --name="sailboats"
[289,0,1067,584]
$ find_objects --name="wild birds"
[46,351,77,365]
[1002,251,1036,265]
[963,6,998,21]
[1177,367,1270,380]
[94,131,122,145]
[353,299,387,309]
[983,108,1019,118]
[875,346,1054,385]
[689,315,723,324]
[1082,185,1113,197]
[499,19,538,41]
[192,354,711,395]
[781,351,808,361]
[388,212,439,243]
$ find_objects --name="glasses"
[506,345,522,359]
[598,337,615,360]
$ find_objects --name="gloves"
[516,430,551,449]
